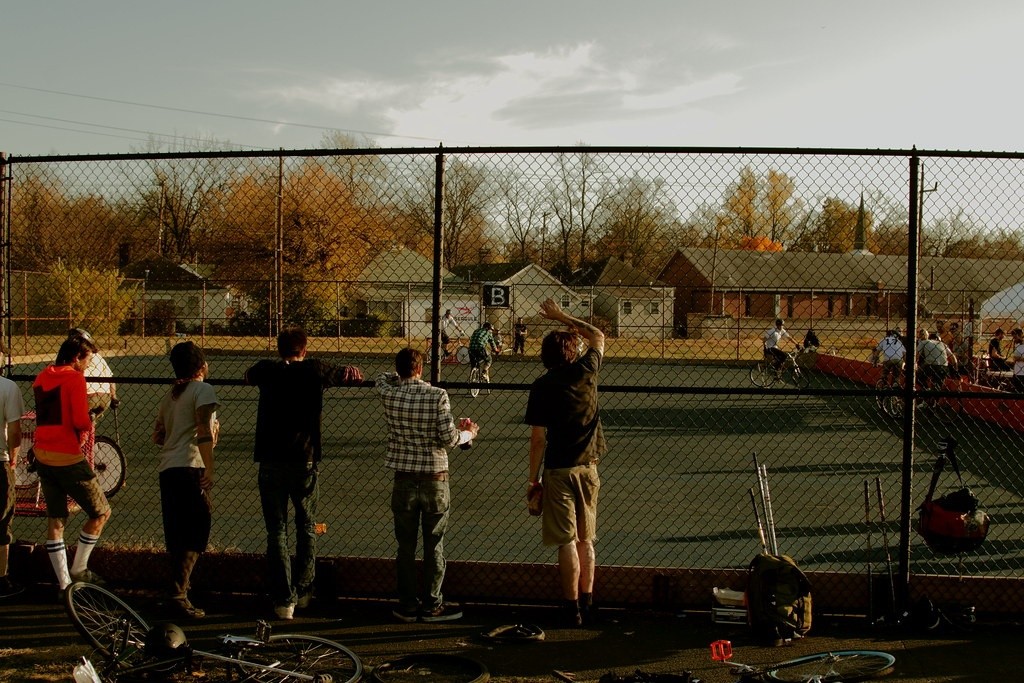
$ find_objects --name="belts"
[394,471,449,481]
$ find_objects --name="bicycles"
[469,352,498,397]
[66,581,364,683]
[749,344,810,388]
[553,641,897,683]
[10,431,126,498]
[874,357,963,423]
[426,331,470,365]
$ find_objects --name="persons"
[916,329,928,345]
[917,333,958,391]
[989,328,1006,371]
[153,339,220,620]
[32,335,112,595]
[376,348,479,624]
[872,330,906,390]
[244,328,366,621]
[763,318,798,375]
[468,322,500,378]
[0,343,26,596]
[442,309,459,355]
[1011,328,1024,391]
[67,328,119,420]
[936,320,968,373]
[513,317,527,353]
[524,299,605,626]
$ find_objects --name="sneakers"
[547,605,583,628]
[421,607,462,621]
[393,605,417,622]
[161,597,205,618]
[297,593,311,607]
[275,604,295,619]
[1,576,27,598]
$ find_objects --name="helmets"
[446,309,452,314]
[483,322,494,329]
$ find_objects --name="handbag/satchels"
[914,439,992,555]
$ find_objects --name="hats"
[170,341,205,378]
[929,334,941,341]
[887,329,901,339]
[776,319,784,325]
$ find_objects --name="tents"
[980,282,1024,336]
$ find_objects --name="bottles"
[459,417,472,449]
[529,484,545,515]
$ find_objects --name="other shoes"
[57,590,69,605]
[481,372,487,379]
[446,351,452,355]
[68,568,106,584]
